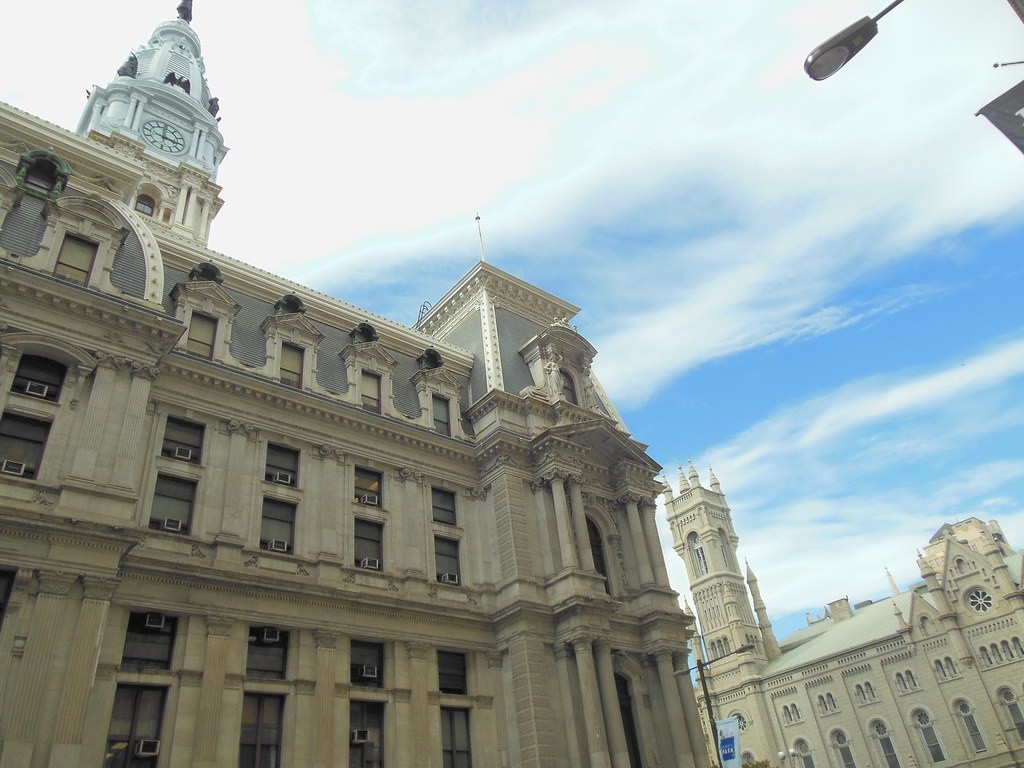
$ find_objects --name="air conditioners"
[23,382,48,398]
[359,557,379,570]
[159,518,182,533]
[259,628,280,644]
[351,729,368,745]
[143,614,165,629]
[1,460,25,476]
[172,446,191,461]
[273,472,290,485]
[135,739,160,757]
[441,573,458,585]
[357,665,377,678]
[268,539,287,552]
[361,494,378,505]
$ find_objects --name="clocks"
[138,118,190,157]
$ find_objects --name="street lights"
[671,643,756,768]
